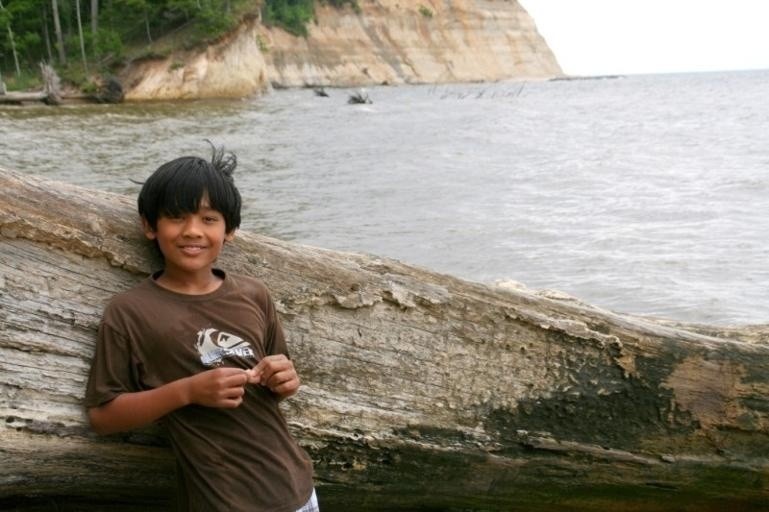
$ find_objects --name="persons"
[83,137,320,512]
